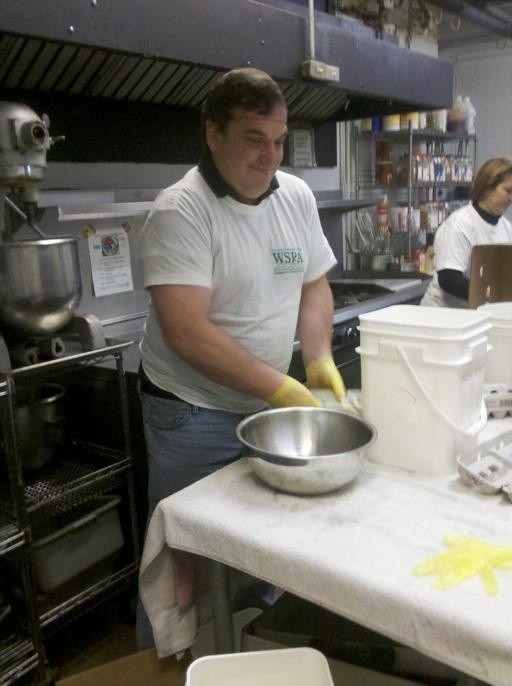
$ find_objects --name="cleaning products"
[394,154,472,181]
[376,194,471,231]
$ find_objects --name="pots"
[15,383,67,474]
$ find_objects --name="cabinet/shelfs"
[351,118,478,273]
[0,335,140,686]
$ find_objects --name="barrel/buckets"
[448,96,468,134]
[463,96,476,134]
[477,302,512,385]
[355,303,493,476]
[184,646,336,686]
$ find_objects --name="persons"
[135,67,347,649]
[421,158,512,309]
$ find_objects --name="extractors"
[0,1,457,140]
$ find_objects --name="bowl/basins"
[359,255,390,272]
[234,406,378,497]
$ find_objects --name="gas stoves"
[294,279,396,359]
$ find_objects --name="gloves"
[305,356,347,401]
[266,376,320,408]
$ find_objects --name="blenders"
[0,100,103,372]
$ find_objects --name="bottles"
[419,200,448,229]
[415,152,472,183]
[378,194,389,234]
[451,94,478,134]
